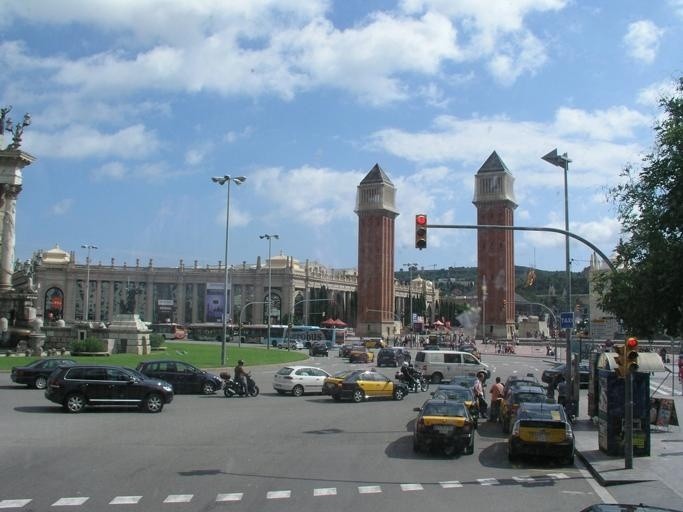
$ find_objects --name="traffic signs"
[559,311,575,329]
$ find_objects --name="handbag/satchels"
[479,396,487,413]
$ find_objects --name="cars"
[338,343,357,357]
[116,366,173,390]
[272,365,332,396]
[427,385,479,430]
[277,338,304,350]
[412,399,475,456]
[459,345,480,359]
[508,402,575,464]
[322,370,407,401]
[497,371,548,433]
[423,345,440,350]
[448,374,481,403]
[10,357,79,389]
[349,345,374,363]
[542,361,589,389]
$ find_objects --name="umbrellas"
[359,329,554,356]
[322,318,348,343]
[433,319,443,326]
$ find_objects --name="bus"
[264,325,322,348]
[189,322,233,342]
[150,323,186,340]
[321,328,344,343]
[239,325,268,344]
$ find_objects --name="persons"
[638,345,682,384]
[234,359,251,398]
[400,359,568,424]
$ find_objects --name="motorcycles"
[219,370,260,398]
[394,370,429,393]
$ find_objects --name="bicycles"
[558,397,576,427]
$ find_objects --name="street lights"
[210,173,247,367]
[259,233,279,348]
[80,243,99,320]
[403,262,418,327]
[541,145,572,387]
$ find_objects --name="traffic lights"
[614,346,625,376]
[415,214,427,251]
[624,336,639,374]
[504,300,506,307]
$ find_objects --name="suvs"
[377,347,404,367]
[309,343,328,357]
[44,365,174,416]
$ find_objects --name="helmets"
[238,360,244,363]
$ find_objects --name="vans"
[414,350,490,387]
[135,362,223,397]
